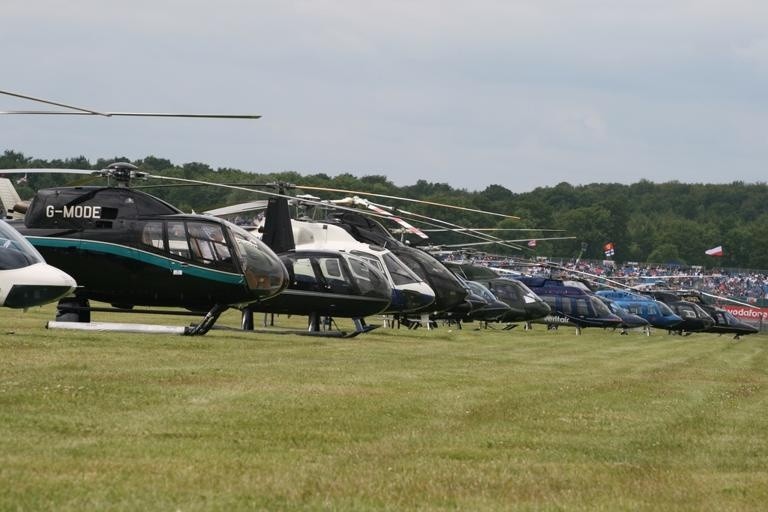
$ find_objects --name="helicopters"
[0,89,760,344]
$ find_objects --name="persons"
[200,227,231,267]
[470,256,768,300]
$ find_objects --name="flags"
[528,240,536,246]
[704,245,722,256]
[603,242,614,257]
